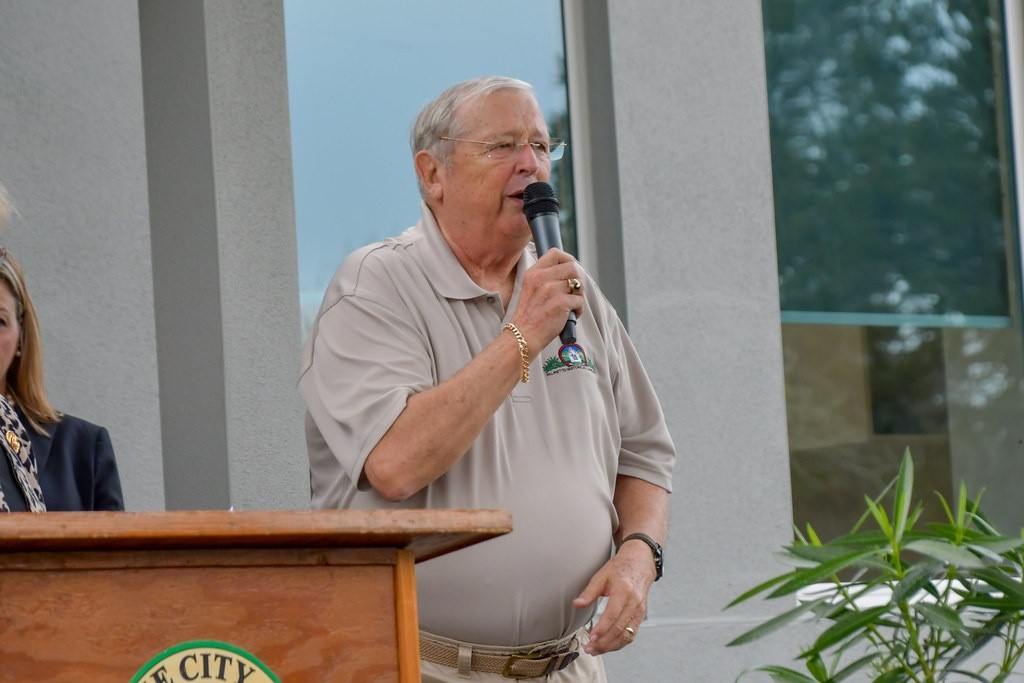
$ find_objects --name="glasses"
[439,136,567,161]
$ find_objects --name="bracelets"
[504,322,530,384]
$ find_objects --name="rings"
[625,627,634,635]
[567,278,581,294]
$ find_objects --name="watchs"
[615,533,664,582]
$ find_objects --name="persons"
[294,74,674,683]
[0,244,127,513]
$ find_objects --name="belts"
[418,620,594,679]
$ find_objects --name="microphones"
[520,182,577,345]
